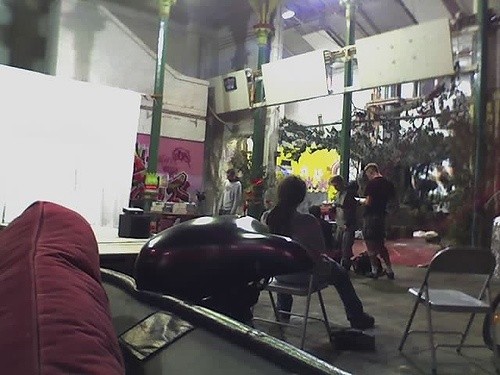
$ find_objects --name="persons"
[265,176,375,330]
[330,175,359,270]
[363,163,394,281]
[222,169,242,215]
[260,202,273,228]
[309,205,332,251]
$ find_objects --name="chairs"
[399,246,497,375]
[252,234,334,353]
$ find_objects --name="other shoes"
[350,312,376,330]
[383,268,395,279]
[365,271,379,279]
